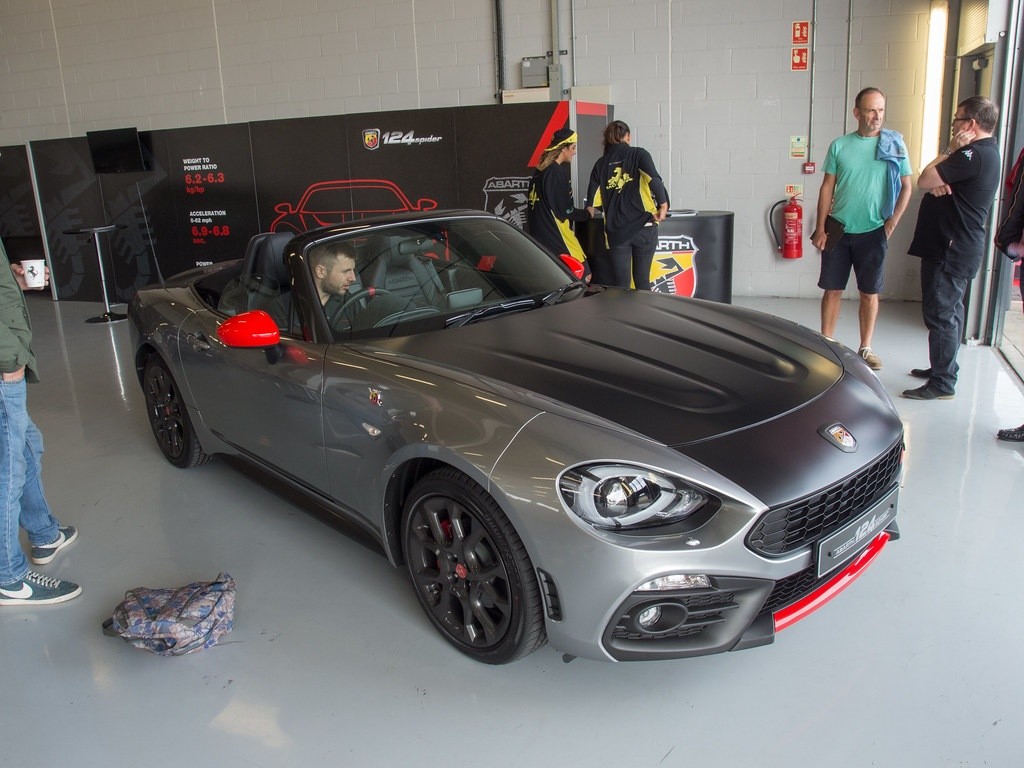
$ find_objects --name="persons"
[901,95,1001,400]
[586,121,670,290]
[264,237,367,339]
[811,83,913,375]
[0,233,88,604]
[523,128,597,286]
[993,144,1024,441]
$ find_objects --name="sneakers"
[858,346,882,370]
[911,368,932,379]
[31,525,79,565]
[0,570,82,606]
[902,383,954,400]
[996,424,1024,442]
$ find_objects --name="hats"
[542,128,577,152]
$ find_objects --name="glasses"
[953,114,980,125]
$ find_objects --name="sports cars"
[127,206,909,668]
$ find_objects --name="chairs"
[219,231,307,338]
[370,234,455,315]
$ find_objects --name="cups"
[20,259,45,288]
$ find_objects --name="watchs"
[941,146,953,156]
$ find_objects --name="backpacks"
[102,572,237,658]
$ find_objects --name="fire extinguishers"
[770,193,804,259]
